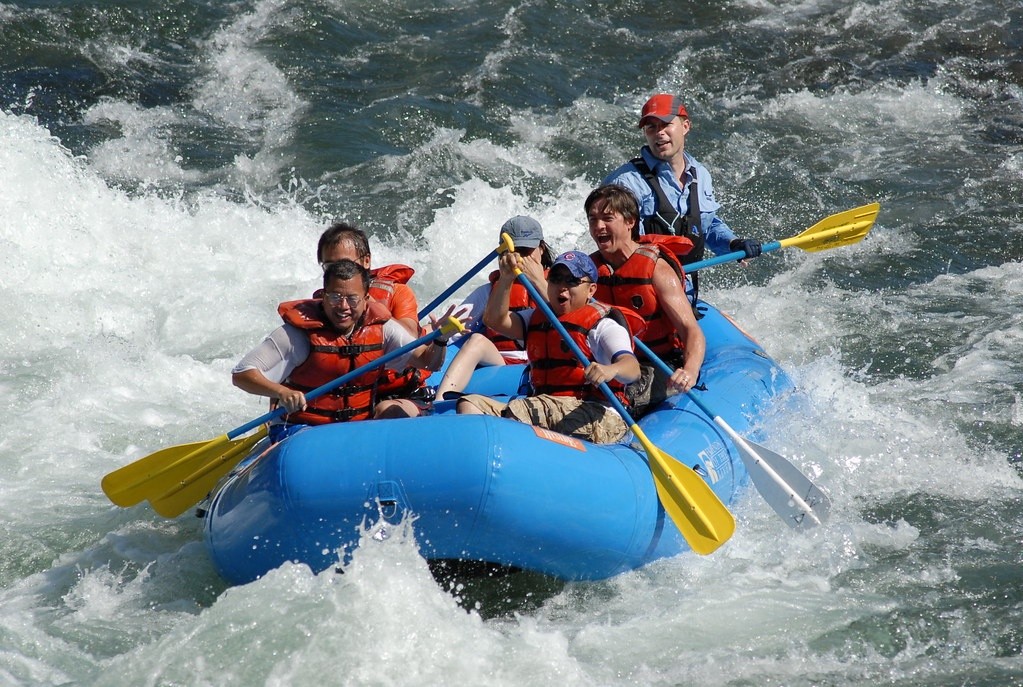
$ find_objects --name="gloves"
[730,239,762,263]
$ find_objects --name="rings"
[683,378,688,383]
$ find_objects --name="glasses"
[514,246,541,256]
[550,271,588,287]
[325,292,366,308]
[322,255,363,271]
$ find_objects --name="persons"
[585,184,705,394]
[230,221,473,446]
[456,252,641,447]
[434,215,553,402]
[602,94,763,322]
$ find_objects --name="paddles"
[102,315,462,509]
[684,202,880,280]
[582,287,832,535]
[148,245,501,520]
[499,223,734,555]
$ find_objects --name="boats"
[194,276,793,584]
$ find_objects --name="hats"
[638,94,688,129]
[499,215,544,249]
[548,251,597,282]
